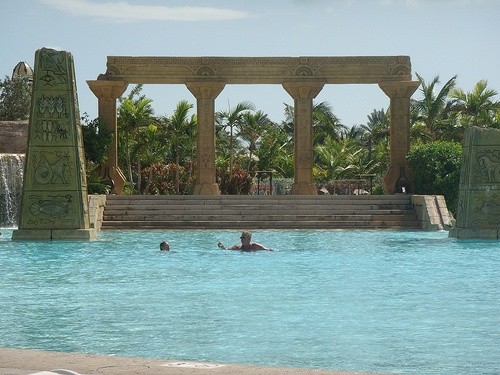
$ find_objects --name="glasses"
[239,236,245,239]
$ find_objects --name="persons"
[218,231,273,251]
[159,240,171,252]
